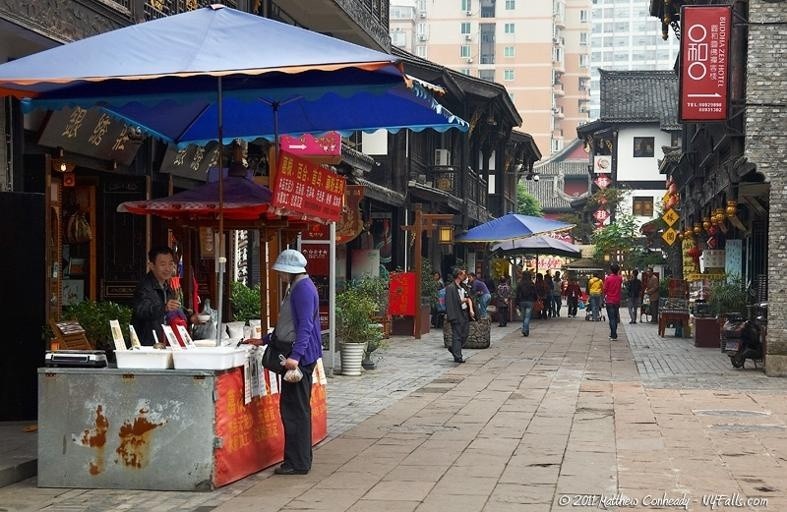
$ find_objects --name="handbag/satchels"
[262,332,296,375]
[578,299,585,310]
[533,294,544,314]
[643,292,650,305]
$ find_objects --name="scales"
[45,349,108,368]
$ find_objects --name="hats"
[271,249,307,274]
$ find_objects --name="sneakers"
[608,335,616,340]
[522,329,529,336]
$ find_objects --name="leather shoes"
[275,462,309,474]
[448,346,465,363]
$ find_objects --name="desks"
[31,356,330,496]
[691,313,721,350]
[655,310,688,340]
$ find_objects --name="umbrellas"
[0,4,412,348]
[491,236,580,273]
[123,164,272,318]
[97,79,470,319]
[453,211,577,287]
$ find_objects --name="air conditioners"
[463,34,471,40]
[434,146,451,171]
[419,13,425,19]
[464,11,472,16]
[334,288,370,378]
[465,58,472,63]
[418,36,426,42]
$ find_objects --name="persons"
[166,246,201,326]
[429,262,660,363]
[242,249,323,474]
[131,244,180,345]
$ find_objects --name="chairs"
[47,313,93,352]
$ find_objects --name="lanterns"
[662,183,736,261]
[438,222,455,247]
